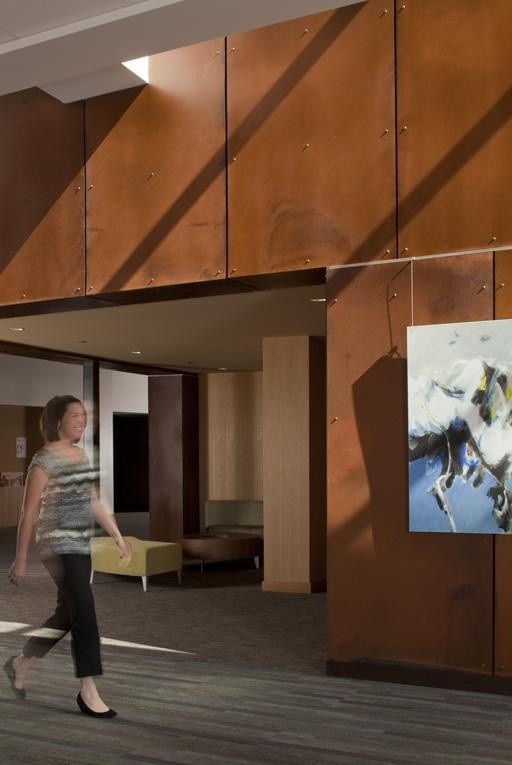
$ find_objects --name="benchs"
[201,499,264,534]
[86,537,185,593]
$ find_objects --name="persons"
[2,394,133,720]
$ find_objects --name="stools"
[176,531,262,574]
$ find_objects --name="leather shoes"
[77,691,117,719]
[4,656,25,699]
